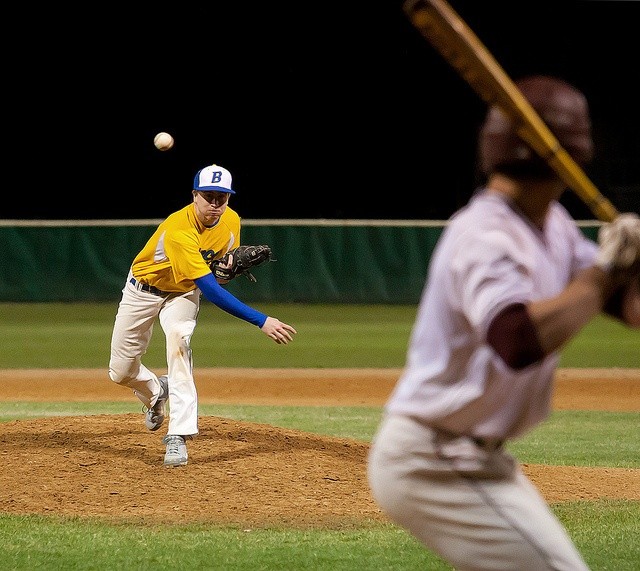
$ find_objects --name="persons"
[107,164,297,468]
[366,78,640,570]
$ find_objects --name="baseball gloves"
[210,245,271,282]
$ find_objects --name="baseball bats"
[403,0,621,222]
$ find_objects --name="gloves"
[594,212,639,274]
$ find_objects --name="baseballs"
[154,132,174,152]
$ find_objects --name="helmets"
[479,78,594,173]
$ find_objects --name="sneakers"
[142,375,169,431]
[161,436,188,466]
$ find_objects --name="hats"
[193,164,237,194]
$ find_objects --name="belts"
[130,278,167,296]
